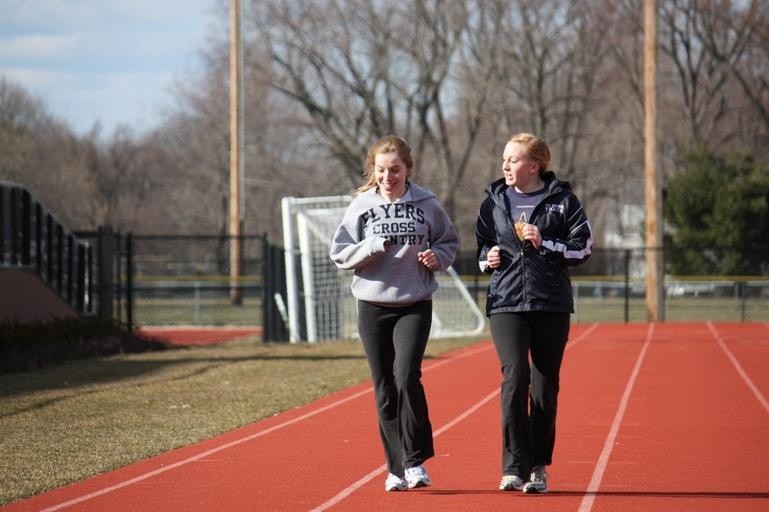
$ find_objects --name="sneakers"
[499,467,548,492]
[385,464,431,491]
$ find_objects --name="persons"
[331,135,461,489]
[477,135,597,491]
